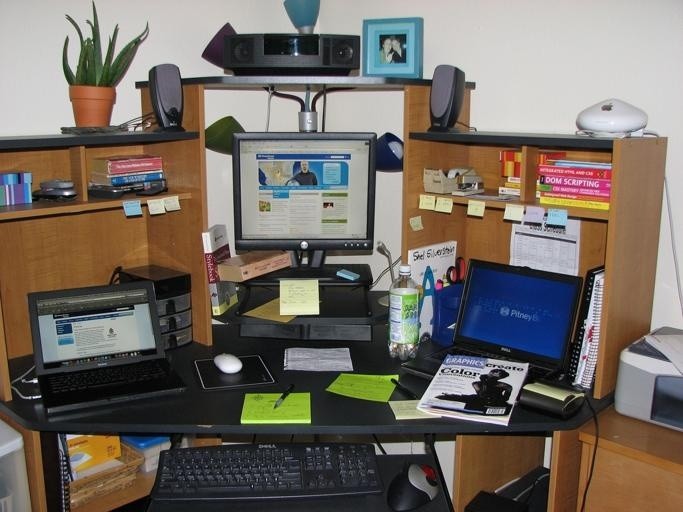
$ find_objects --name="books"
[201,224,239,317]
[498,149,524,197]
[567,264,605,389]
[535,150,612,211]
[217,251,293,283]
[415,353,530,427]
[89,153,167,194]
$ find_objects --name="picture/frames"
[363,17,427,78]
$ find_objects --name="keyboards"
[150,442,383,501]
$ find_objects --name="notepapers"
[337,269,360,281]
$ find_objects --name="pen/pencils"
[391,378,416,399]
[273,383,294,408]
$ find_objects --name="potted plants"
[53,1,150,128]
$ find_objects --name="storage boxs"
[120,433,192,475]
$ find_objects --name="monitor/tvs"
[232,131,377,287]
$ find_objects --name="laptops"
[400,258,584,380]
[27,281,189,415]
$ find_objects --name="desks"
[0,334,614,512]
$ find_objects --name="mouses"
[387,463,440,512]
[214,353,243,374]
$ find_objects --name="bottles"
[388,265,419,361]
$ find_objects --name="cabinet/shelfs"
[579,400,683,512]
[131,75,474,347]
[0,130,212,401]
[406,127,667,401]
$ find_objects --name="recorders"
[223,32,360,76]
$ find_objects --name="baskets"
[70,440,145,510]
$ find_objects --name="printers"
[615,326,683,432]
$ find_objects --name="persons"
[282,160,317,186]
[379,37,393,64]
[434,368,513,415]
[390,37,406,63]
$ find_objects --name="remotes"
[88,184,124,200]
[40,179,74,189]
[32,188,78,201]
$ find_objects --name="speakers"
[149,64,185,132]
[427,65,465,132]
